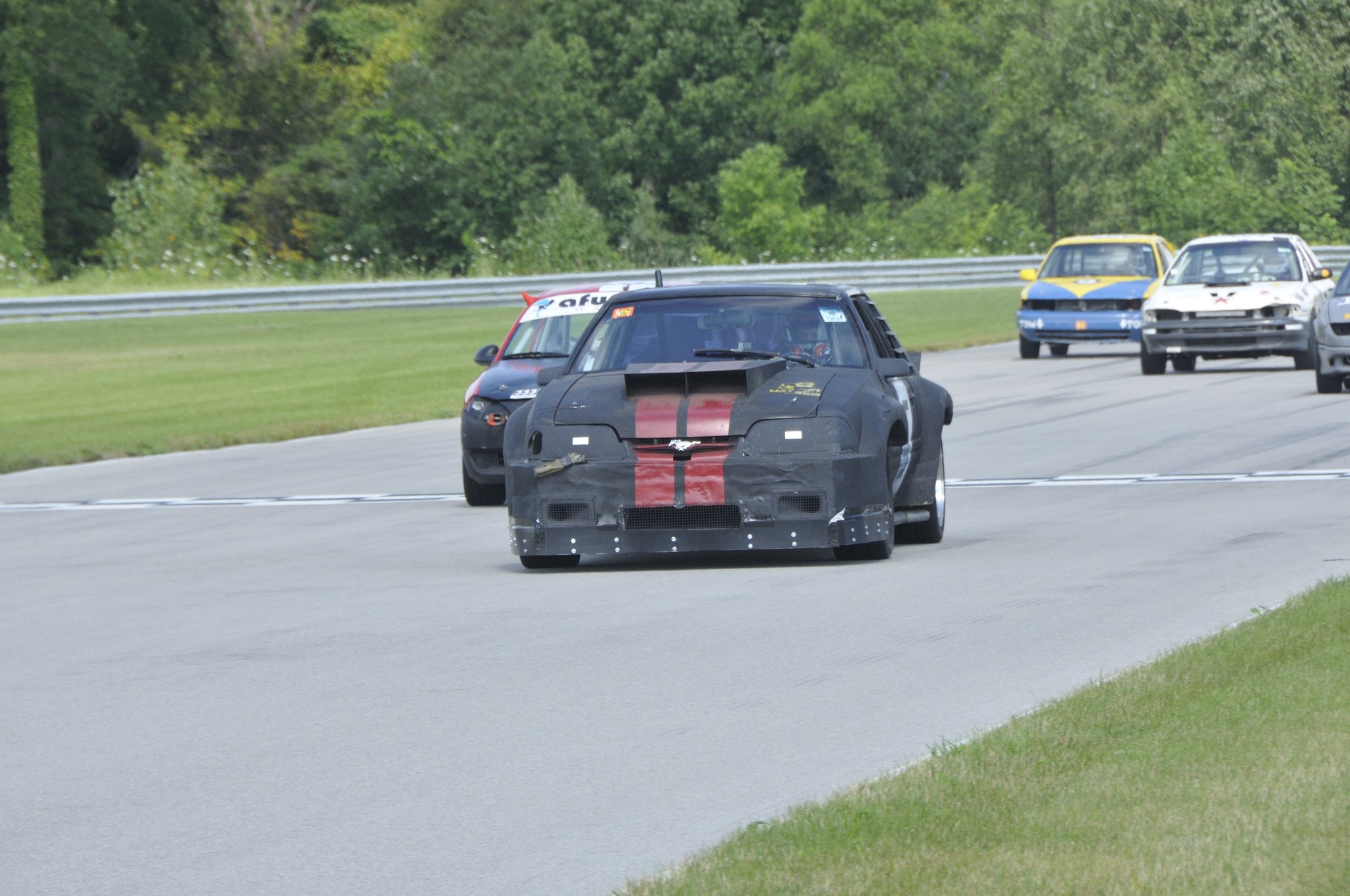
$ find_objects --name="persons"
[1127,249,1147,273]
[1262,248,1292,280]
[785,310,832,365]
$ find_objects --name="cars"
[1016,234,1179,359]
[461,281,695,508]
[1313,260,1350,395]
[1139,233,1335,375]
[503,282,954,568]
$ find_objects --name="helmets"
[1127,249,1151,274]
[786,309,831,351]
[1260,247,1290,275]
